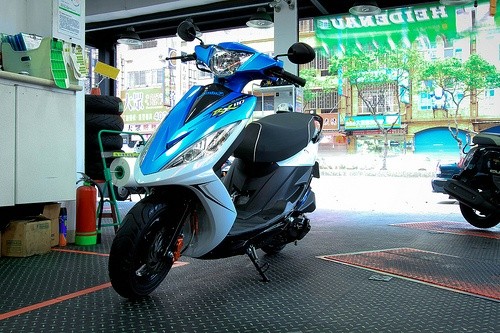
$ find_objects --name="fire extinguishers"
[75,171,104,246]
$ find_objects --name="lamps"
[246,7,274,28]
[116,26,142,45]
[349,0,382,16]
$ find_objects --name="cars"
[431,162,463,194]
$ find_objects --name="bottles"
[59,207,67,246]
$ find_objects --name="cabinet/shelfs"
[0,77,83,206]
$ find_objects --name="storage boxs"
[2,37,52,79]
[0,213,52,258]
[43,204,61,247]
[86,45,99,92]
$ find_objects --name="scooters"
[108,21,325,300]
[443,125,500,229]
